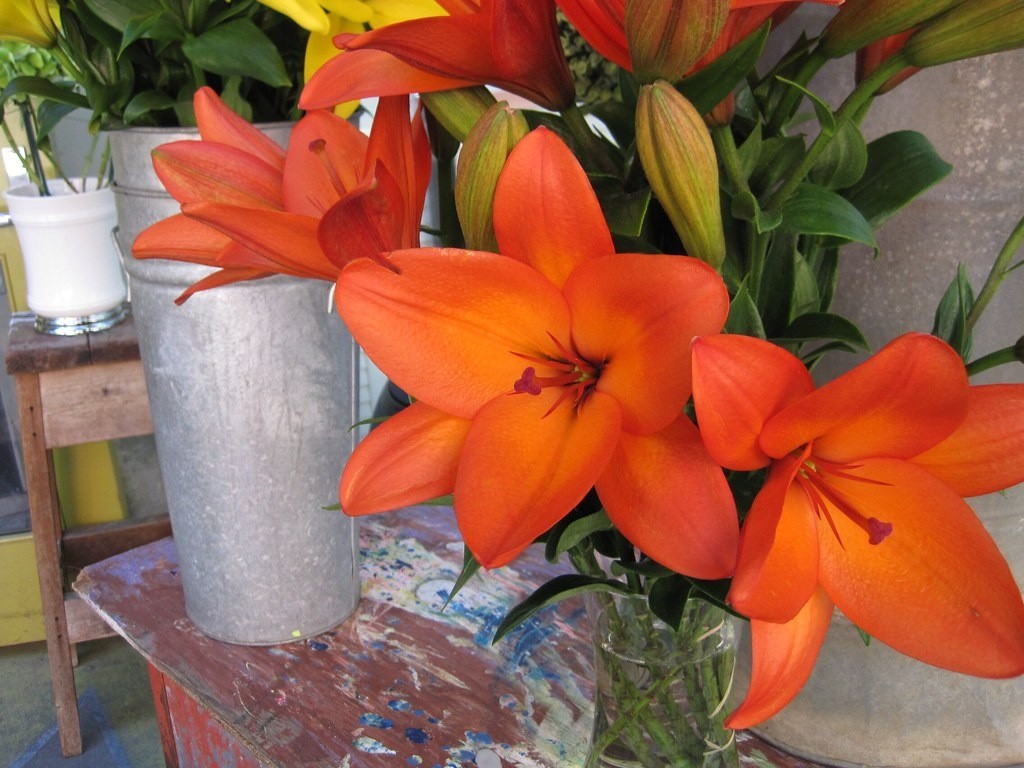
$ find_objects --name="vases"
[41,75,112,177]
[106,129,362,644]
[571,554,745,768]
[3,174,128,336]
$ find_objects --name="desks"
[69,493,832,766]
[3,304,155,757]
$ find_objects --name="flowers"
[0,0,1024,768]
